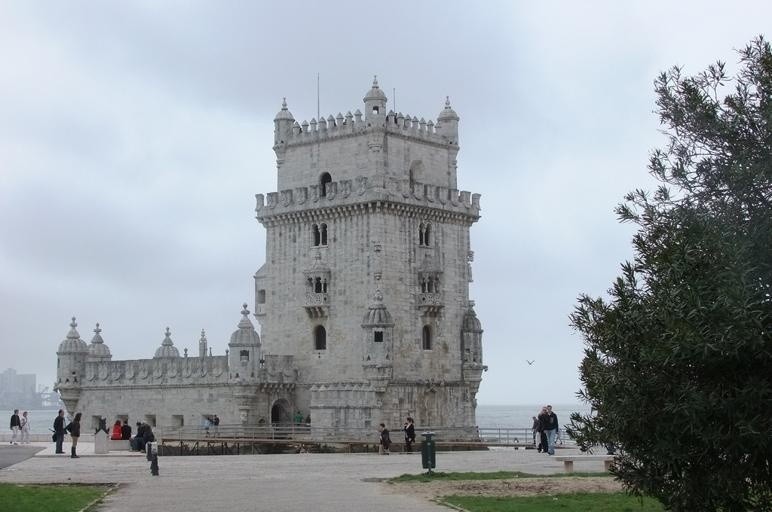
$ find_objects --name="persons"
[111,419,122,439]
[53,409,66,453]
[138,424,154,452]
[555,425,562,444]
[67,412,83,458]
[405,416,416,452]
[213,414,221,438]
[120,421,131,440]
[305,415,310,431]
[532,416,537,444]
[544,404,558,454]
[205,416,211,438]
[375,422,389,455]
[130,421,143,451]
[21,411,32,444]
[10,409,22,444]
[538,406,548,453]
[294,410,303,426]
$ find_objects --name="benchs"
[555,455,615,473]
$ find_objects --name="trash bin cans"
[421,431,436,474]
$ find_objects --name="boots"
[71,447,80,458]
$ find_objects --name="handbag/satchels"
[52,434,57,442]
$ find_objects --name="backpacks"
[64,423,72,434]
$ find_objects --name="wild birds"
[525,358,535,365]
